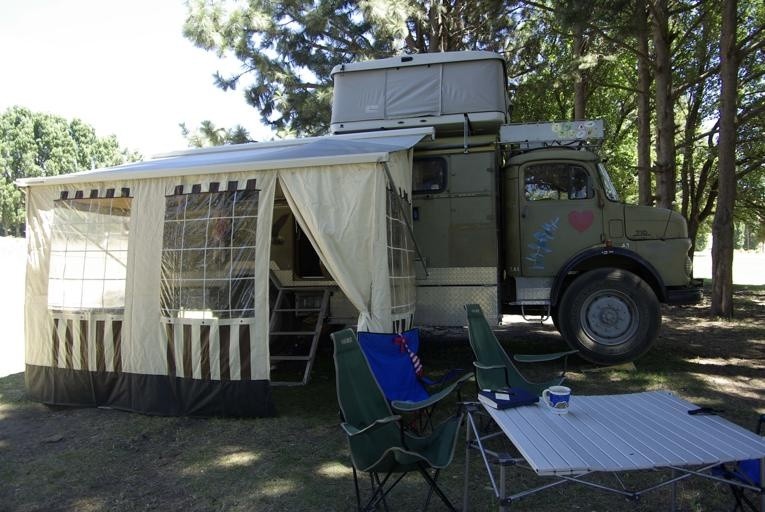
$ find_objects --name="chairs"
[463,303,581,433]
[330,328,477,510]
[356,327,471,440]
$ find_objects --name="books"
[479,385,537,409]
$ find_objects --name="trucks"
[160,132,703,365]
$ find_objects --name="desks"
[456,387,765,511]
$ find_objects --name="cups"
[542,386,570,413]
